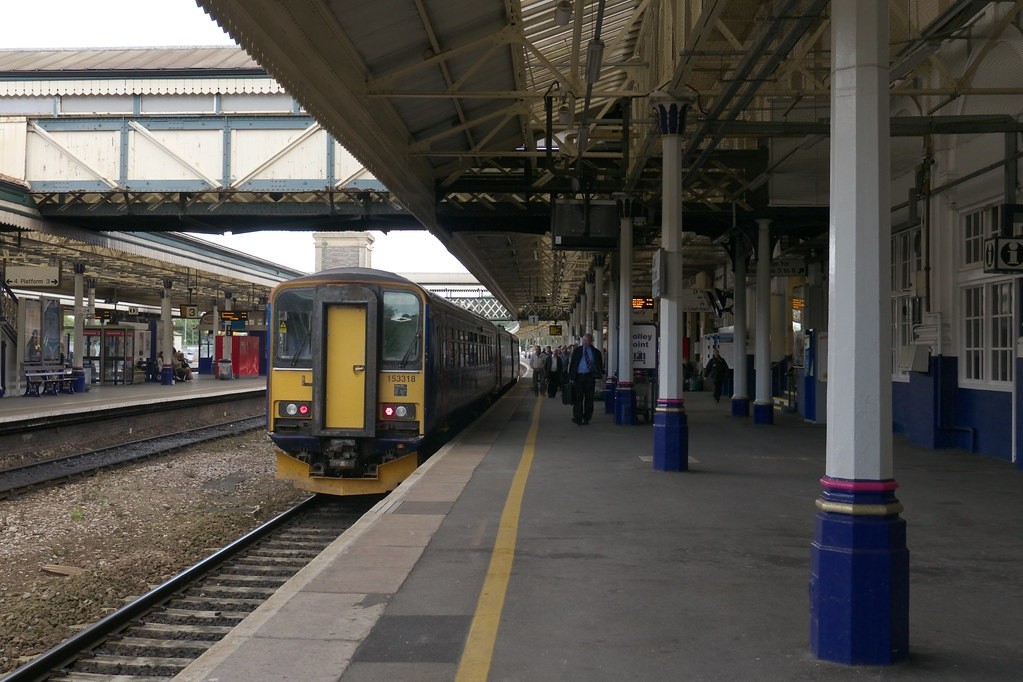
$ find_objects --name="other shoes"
[582,417,590,426]
[571,417,583,425]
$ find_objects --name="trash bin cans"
[218,359,232,380]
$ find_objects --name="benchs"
[24,364,78,399]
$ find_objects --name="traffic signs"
[3,265,62,289]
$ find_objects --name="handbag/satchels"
[560,382,579,406]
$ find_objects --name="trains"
[265,268,520,496]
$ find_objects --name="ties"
[583,347,597,375]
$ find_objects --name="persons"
[703,348,732,405]
[544,348,562,398]
[542,343,578,387]
[156,346,194,383]
[683,358,694,391]
[568,333,602,425]
[530,346,546,398]
[558,345,570,391]
